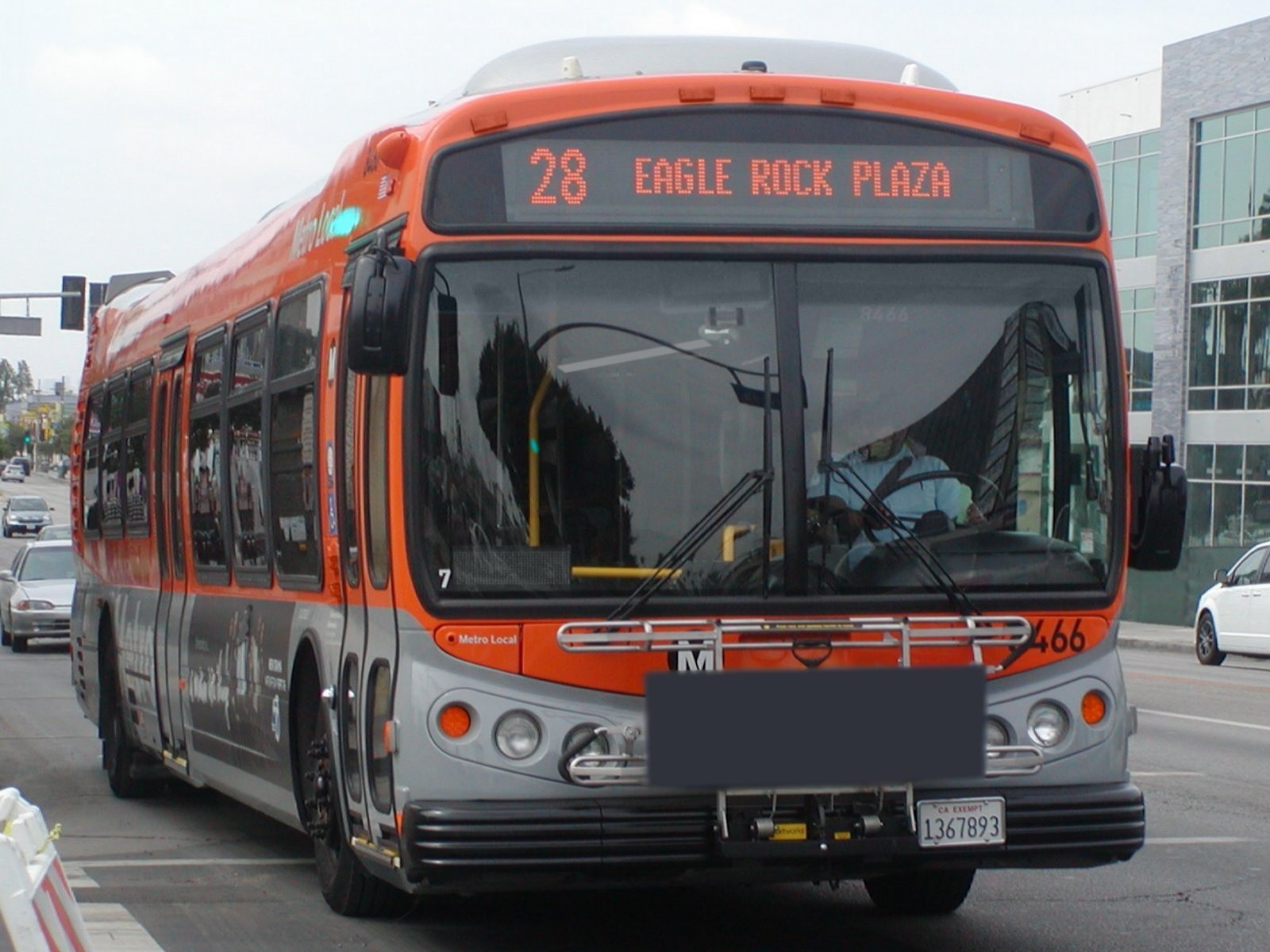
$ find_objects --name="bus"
[73,35,1188,921]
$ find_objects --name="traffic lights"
[21,432,31,444]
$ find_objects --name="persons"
[86,477,145,531]
[805,397,983,572]
[235,469,258,567]
[194,465,226,566]
[302,464,316,542]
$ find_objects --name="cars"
[2,495,55,537]
[0,456,71,483]
[1194,539,1270,665]
[36,524,71,541]
[0,540,76,653]
